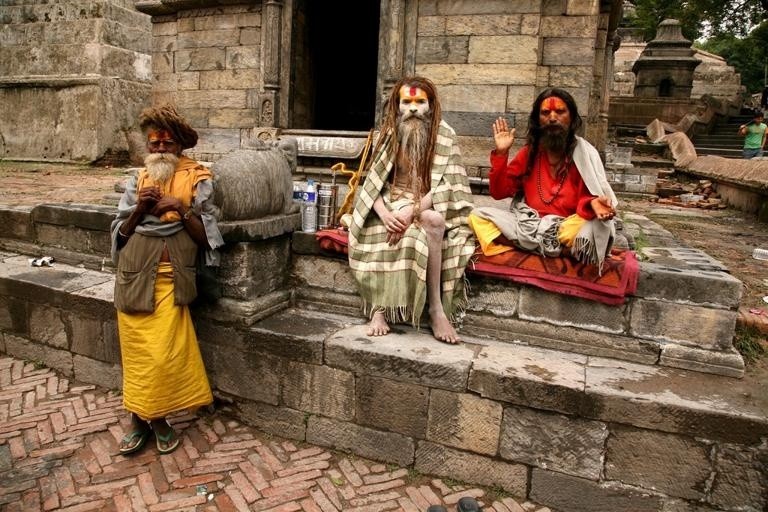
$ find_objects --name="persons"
[488,89,620,279]
[347,73,481,345]
[738,110,768,159]
[108,107,226,455]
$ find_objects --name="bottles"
[315,185,338,230]
[291,182,303,202]
[681,194,704,202]
[752,249,768,262]
[301,180,318,232]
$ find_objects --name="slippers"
[154,420,180,454]
[120,427,151,455]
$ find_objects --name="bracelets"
[182,209,194,220]
